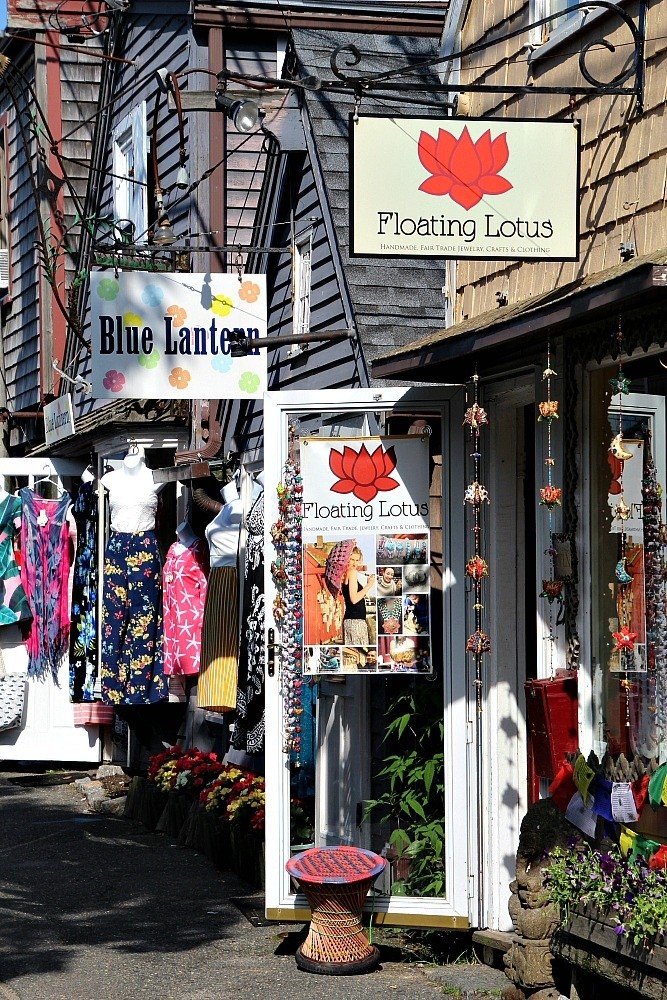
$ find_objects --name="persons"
[377,598,403,635]
[204,469,266,751]
[319,648,339,672]
[389,636,418,671]
[377,568,403,596]
[166,522,207,677]
[405,594,429,631]
[341,548,375,646]
[72,463,102,704]
[100,450,165,705]
[356,651,376,672]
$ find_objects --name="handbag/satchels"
[0,647,26,732]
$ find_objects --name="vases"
[560,891,667,972]
[229,823,261,880]
[167,794,190,836]
[144,784,166,830]
[252,839,265,890]
[207,816,231,869]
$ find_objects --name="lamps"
[213,89,258,133]
[175,167,189,190]
[153,208,178,244]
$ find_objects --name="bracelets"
[377,536,426,562]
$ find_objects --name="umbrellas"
[325,538,356,601]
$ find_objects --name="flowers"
[146,744,316,844]
[536,841,667,957]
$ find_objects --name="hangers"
[13,465,66,502]
